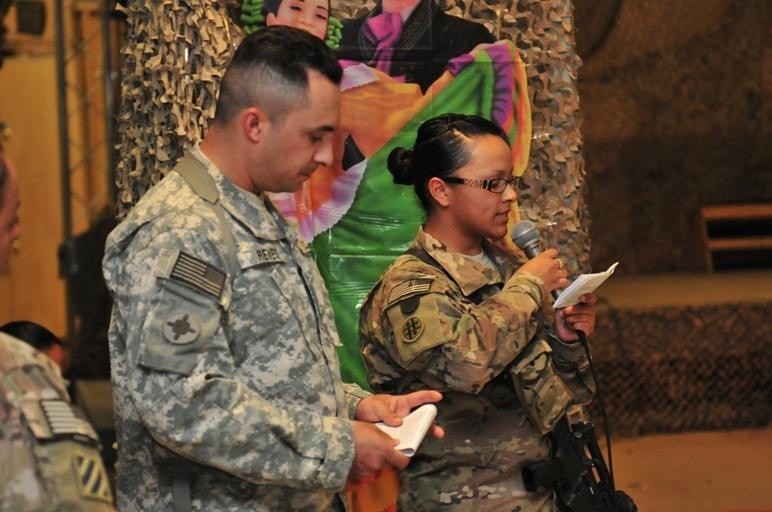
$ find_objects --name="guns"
[519,412,638,511]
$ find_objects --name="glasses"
[438,175,524,195]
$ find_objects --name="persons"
[101,26,445,512]
[335,0,497,171]
[359,112,597,511]
[0,154,22,279]
[1,333,116,511]
[1,319,68,370]
[240,1,533,512]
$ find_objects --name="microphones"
[510,220,591,354]
